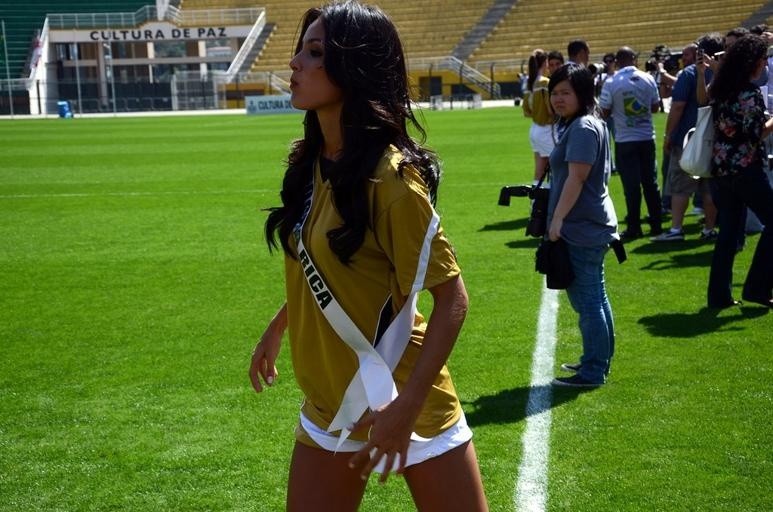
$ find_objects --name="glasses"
[758,55,769,61]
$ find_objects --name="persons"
[547,51,566,75]
[597,48,663,236]
[593,52,617,87]
[707,34,773,310]
[653,43,699,242]
[550,41,599,77]
[695,29,750,243]
[499,62,626,387]
[524,47,561,199]
[250,2,490,512]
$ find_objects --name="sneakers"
[620,229,644,240]
[551,374,602,388]
[742,292,773,308]
[657,230,687,243]
[560,359,609,376]
[645,228,665,237]
[699,230,718,243]
[707,300,739,313]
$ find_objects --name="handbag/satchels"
[535,240,570,274]
[678,105,713,178]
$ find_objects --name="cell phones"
[695,47,704,65]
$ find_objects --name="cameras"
[498,181,552,239]
[589,62,608,74]
[714,51,729,61]
[645,44,670,71]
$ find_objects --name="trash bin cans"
[57,99,74,120]
